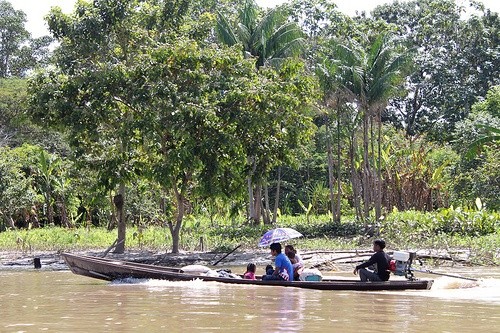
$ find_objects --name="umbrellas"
[257,228,303,247]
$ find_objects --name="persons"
[262,243,294,281]
[285,245,304,281]
[353,239,390,282]
[244,263,256,279]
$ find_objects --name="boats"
[62,250,434,292]
